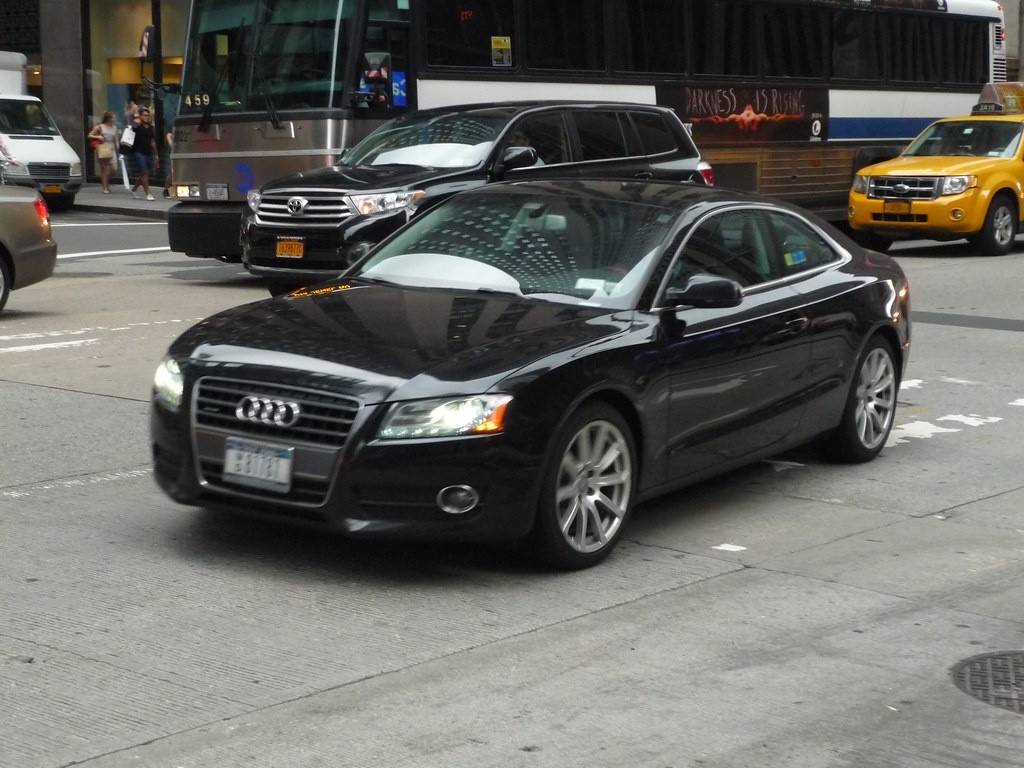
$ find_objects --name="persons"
[132,109,157,201]
[504,128,545,167]
[162,122,175,200]
[87,111,119,194]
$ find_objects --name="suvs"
[241,99,715,297]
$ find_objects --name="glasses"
[141,114,150,117]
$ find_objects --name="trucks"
[0,50,84,212]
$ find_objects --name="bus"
[138,0,1007,263]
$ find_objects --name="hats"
[139,107,151,115]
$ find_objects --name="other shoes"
[102,186,112,193]
[146,193,155,201]
[131,190,141,199]
[163,188,171,198]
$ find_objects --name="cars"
[847,83,1024,256]
[0,185,58,313]
[150,179,912,571]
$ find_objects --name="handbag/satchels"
[120,124,136,147]
[96,137,114,158]
[119,125,134,155]
[90,125,103,149]
